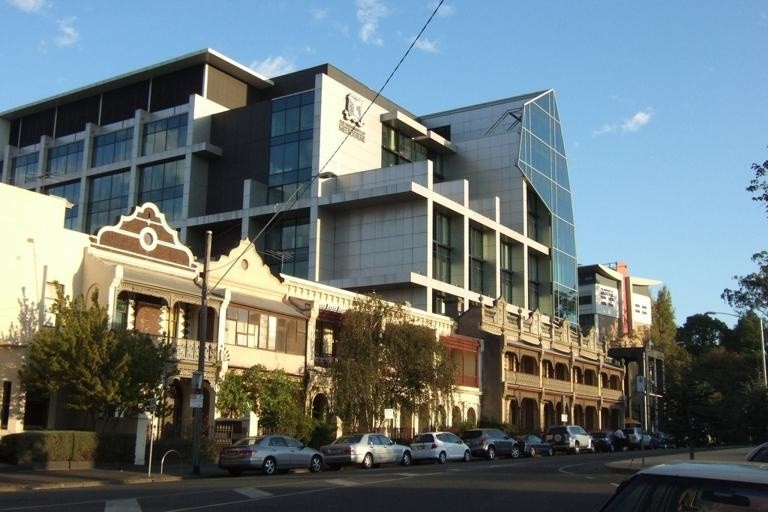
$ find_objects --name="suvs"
[458,427,520,459]
[543,425,596,455]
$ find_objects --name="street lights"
[704,310,768,393]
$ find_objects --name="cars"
[320,433,414,469]
[621,427,654,448]
[744,443,768,461]
[598,458,767,512]
[512,434,553,457]
[648,431,690,448]
[587,430,624,451]
[217,434,325,476]
[410,431,472,463]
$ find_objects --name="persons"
[614,427,627,448]
